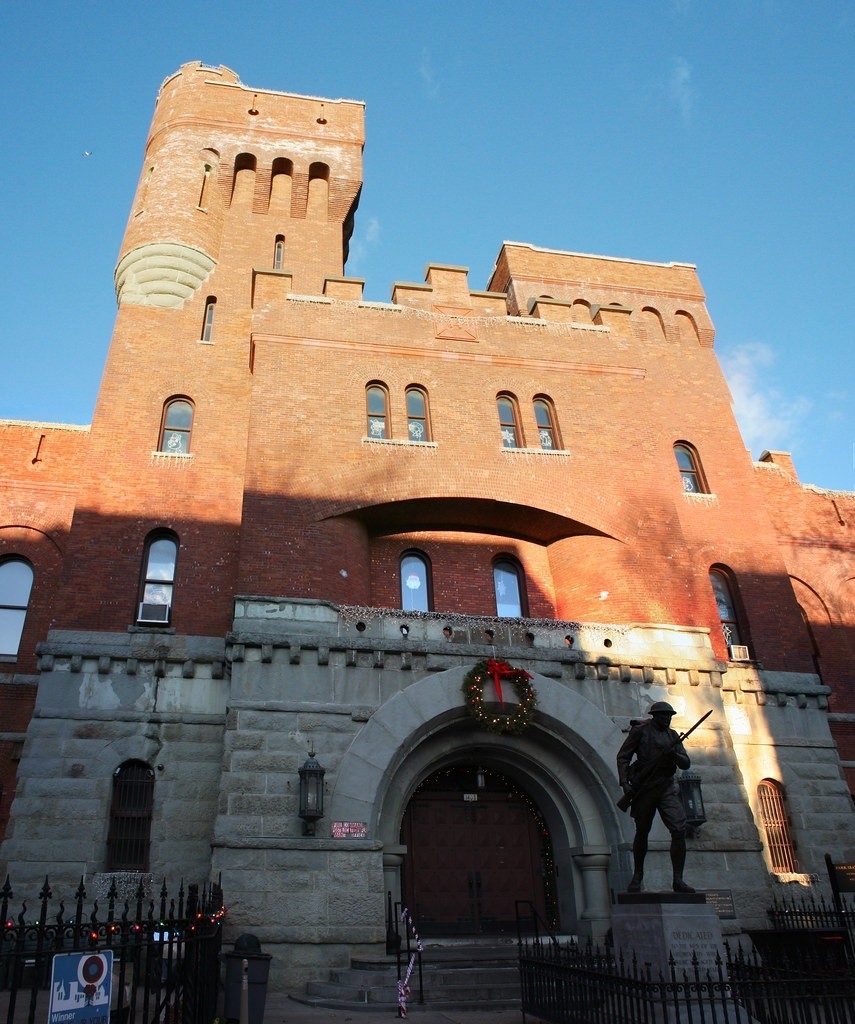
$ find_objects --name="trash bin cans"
[223,933,273,1024]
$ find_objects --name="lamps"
[679,765,709,830]
[297,747,326,836]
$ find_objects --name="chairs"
[0,930,93,990]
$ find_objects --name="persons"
[616,702,695,893]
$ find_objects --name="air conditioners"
[731,644,749,661]
[138,603,169,621]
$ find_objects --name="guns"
[616,709,714,814]
